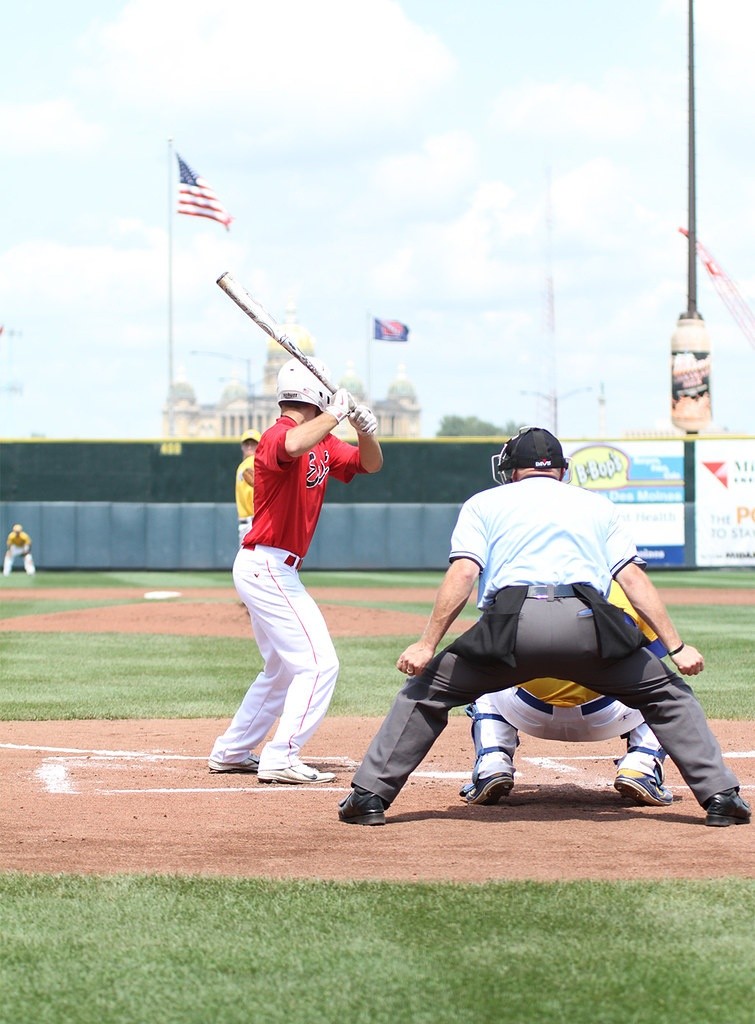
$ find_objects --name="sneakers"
[208,751,259,773]
[259,764,338,782]
[467,773,514,806]
[614,768,673,806]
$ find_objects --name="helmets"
[276,355,334,413]
[14,524,22,531]
[241,430,260,443]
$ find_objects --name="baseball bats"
[214,270,357,412]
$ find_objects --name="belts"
[527,585,576,599]
[243,544,303,569]
[240,520,251,523]
[516,688,614,715]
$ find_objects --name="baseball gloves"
[20,551,28,556]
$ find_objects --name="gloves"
[348,408,378,436]
[324,388,356,424]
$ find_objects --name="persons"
[338,427,751,825]
[3,524,36,577]
[459,556,672,806]
[208,356,383,784]
[235,428,261,615]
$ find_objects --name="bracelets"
[668,640,684,656]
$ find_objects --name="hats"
[499,429,566,469]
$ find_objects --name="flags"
[177,153,236,231]
[374,318,409,342]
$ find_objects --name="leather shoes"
[338,790,385,825]
[706,788,751,826]
